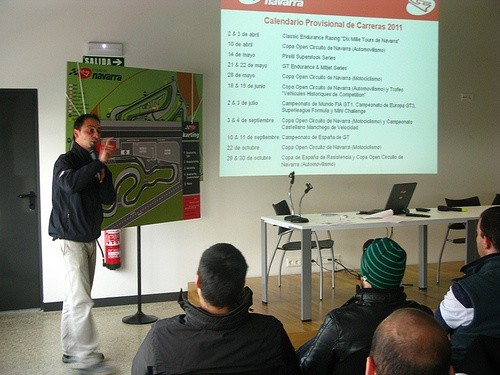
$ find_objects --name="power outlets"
[286,255,340,267]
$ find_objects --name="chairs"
[267,200,335,300]
[436,196,481,283]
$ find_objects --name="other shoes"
[63,352,104,369]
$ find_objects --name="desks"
[261,204,499,322]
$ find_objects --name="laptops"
[358,181,418,213]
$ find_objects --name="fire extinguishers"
[102,228,121,270]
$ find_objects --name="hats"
[360,238,406,290]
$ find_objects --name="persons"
[131,243,304,375]
[49,113,118,375]
[365,308,454,375]
[300,238,437,375]
[435,206,500,375]
